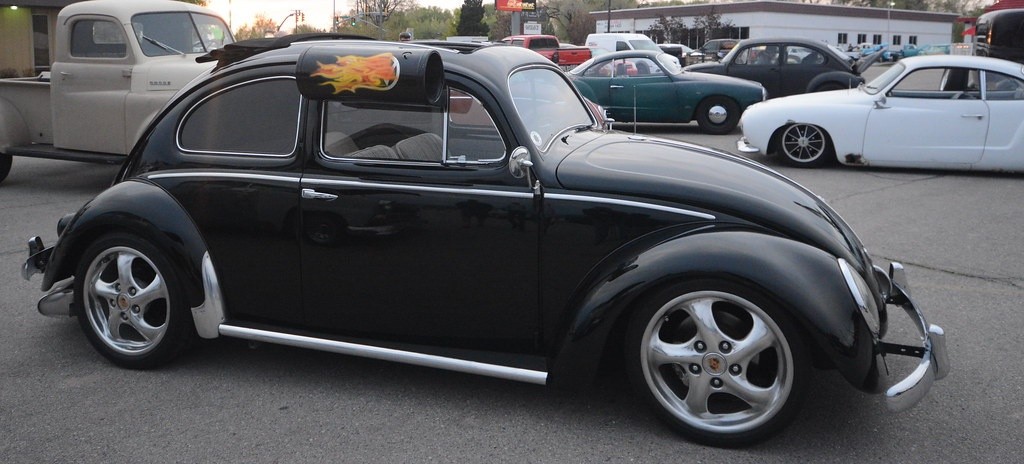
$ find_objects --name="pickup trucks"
[0,0,241,181]
[501,34,592,67]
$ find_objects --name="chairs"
[393,132,453,162]
[346,146,400,160]
[634,60,650,74]
[617,63,628,75]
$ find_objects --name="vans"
[584,32,682,74]
[971,8,1024,86]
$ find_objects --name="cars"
[21,33,949,448]
[735,54,1024,175]
[560,38,951,135]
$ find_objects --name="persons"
[856,47,860,51]
[890,54,899,61]
[617,63,629,77]
[878,46,884,61]
[848,44,853,51]
